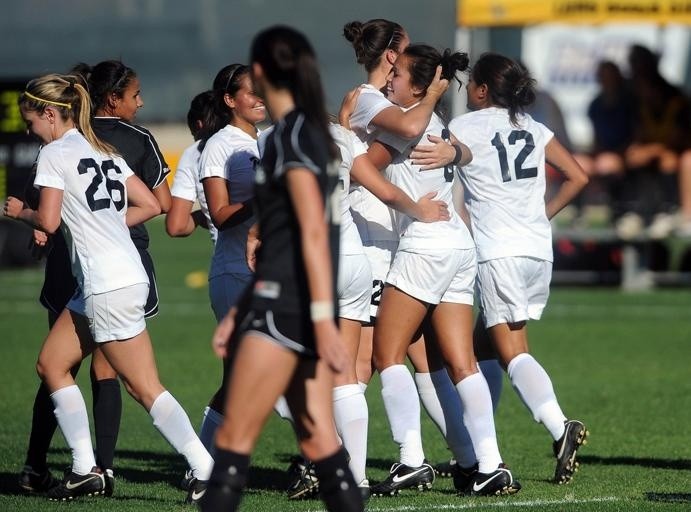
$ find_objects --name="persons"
[2,59,214,501]
[166,20,588,511]
[588,42,691,290]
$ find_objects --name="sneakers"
[18,464,114,501]
[284,446,521,500]
[180,469,211,504]
[551,420,590,485]
